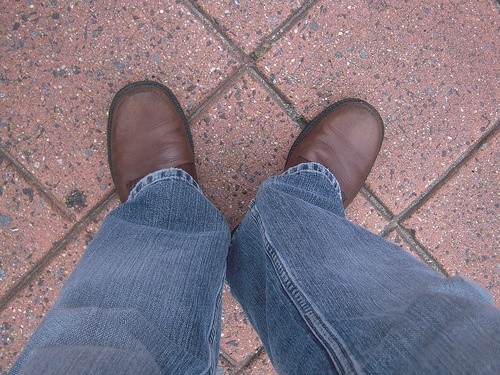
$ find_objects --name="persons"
[4,82,499,375]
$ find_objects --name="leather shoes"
[229,98,384,241]
[105,80,197,204]
